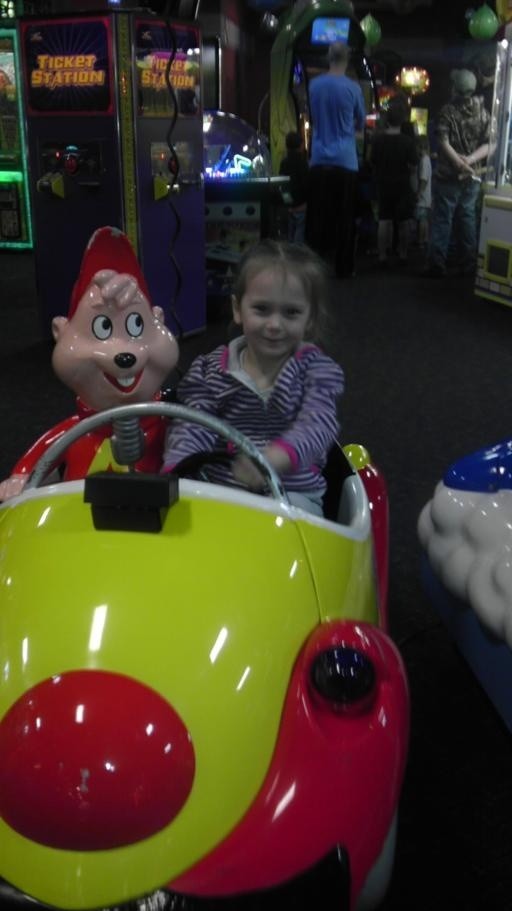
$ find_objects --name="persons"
[417,69,498,280]
[279,131,309,248]
[372,104,419,271]
[302,43,367,285]
[159,237,346,520]
[387,92,418,144]
[407,135,435,259]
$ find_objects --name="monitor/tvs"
[308,16,352,47]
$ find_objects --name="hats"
[449,68,477,92]
